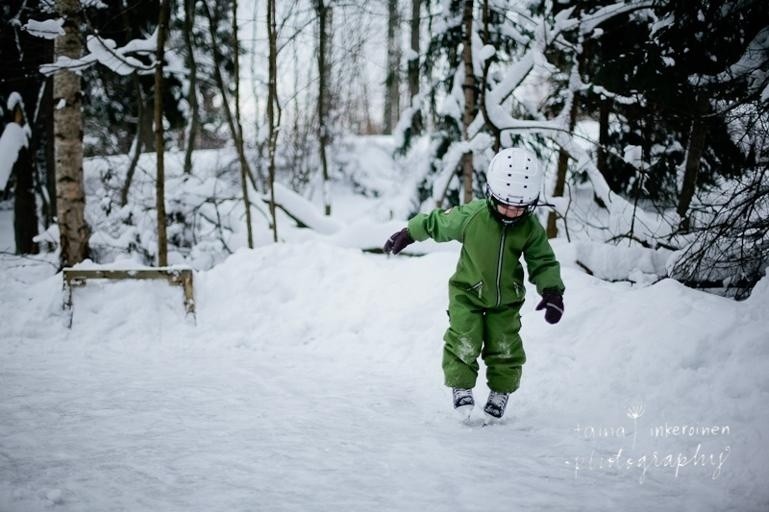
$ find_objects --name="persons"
[386,149,566,420]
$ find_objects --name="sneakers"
[482,388,510,418]
[452,385,475,410]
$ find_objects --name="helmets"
[486,146,542,207]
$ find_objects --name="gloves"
[536,291,565,324]
[383,227,414,255]
